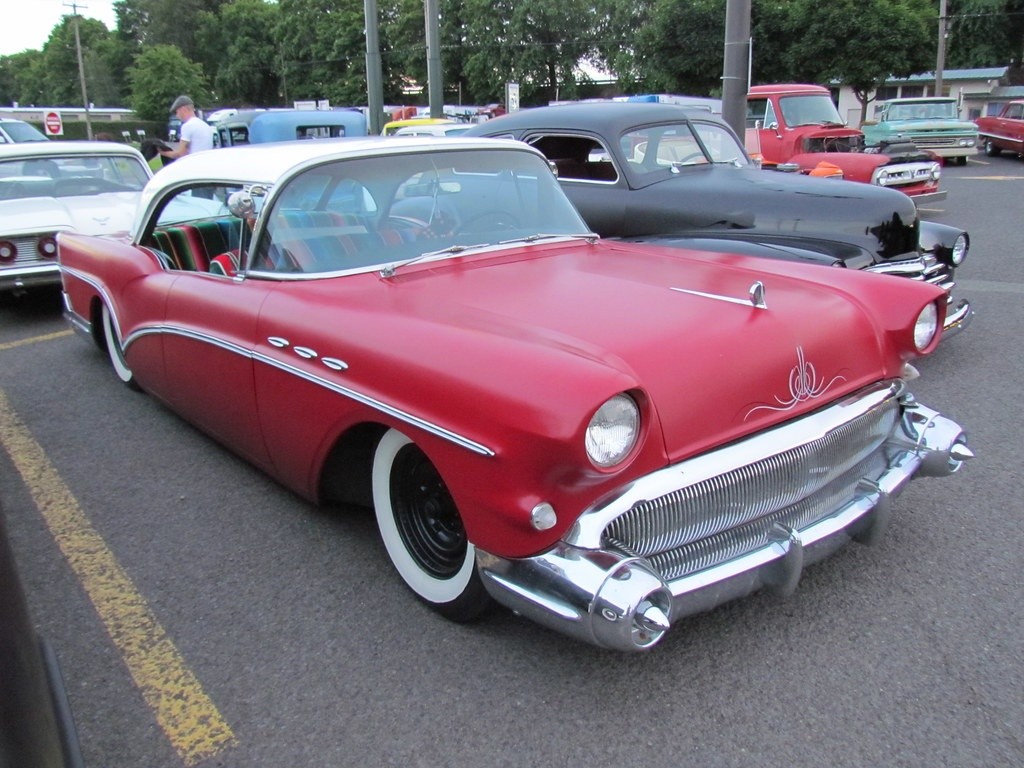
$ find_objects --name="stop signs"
[43,110,64,136]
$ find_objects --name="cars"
[459,92,975,349]
[732,77,1024,210]
[55,139,976,661]
[168,102,509,143]
[0,115,159,294]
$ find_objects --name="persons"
[156,95,213,159]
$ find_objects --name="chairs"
[53,175,100,196]
[0,181,25,201]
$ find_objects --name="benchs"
[207,226,423,278]
[550,158,589,179]
[148,211,371,272]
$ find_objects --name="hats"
[169,95,193,114]
